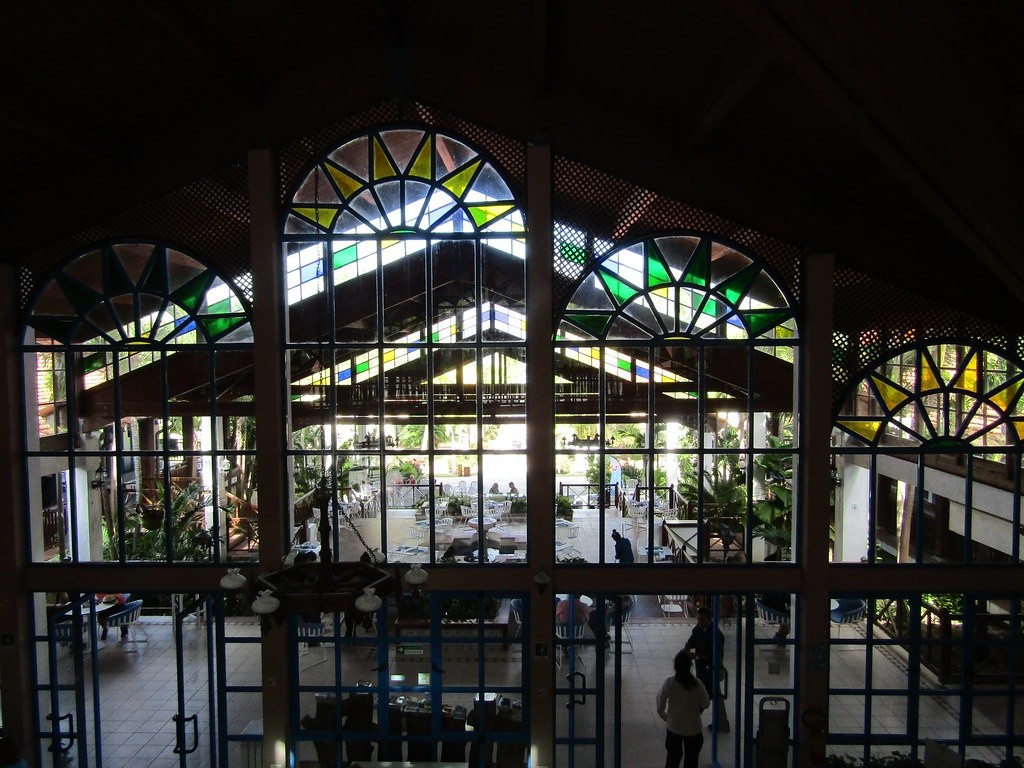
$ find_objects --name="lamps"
[222,479,426,632]
[411,480,512,538]
[352,431,395,448]
[567,434,610,447]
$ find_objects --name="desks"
[830,598,839,611]
[556,594,592,608]
[63,599,115,656]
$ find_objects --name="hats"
[612,529,621,538]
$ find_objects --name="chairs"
[123,480,137,503]
[637,538,656,563]
[295,624,329,671]
[54,621,88,671]
[107,599,148,653]
[511,597,523,642]
[555,624,587,670]
[625,507,647,533]
[830,596,867,650]
[664,509,678,522]
[339,611,377,646]
[557,527,582,558]
[312,505,339,534]
[608,607,633,654]
[754,597,793,642]
[658,595,688,618]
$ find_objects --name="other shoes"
[121,630,128,638]
[604,635,612,650]
[101,630,108,641]
[707,719,730,732]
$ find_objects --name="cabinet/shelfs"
[241,721,264,768]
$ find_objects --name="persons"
[489,483,500,493]
[611,531,634,564]
[587,594,633,653]
[556,593,590,659]
[442,545,458,562]
[762,554,792,644]
[45,592,84,650]
[508,482,518,494]
[94,592,130,640]
[293,546,375,648]
[681,608,731,733]
[655,650,711,768]
[468,539,479,561]
